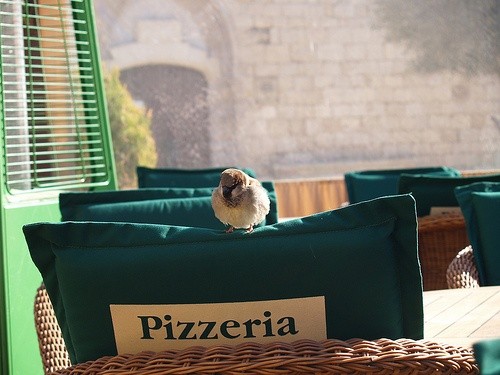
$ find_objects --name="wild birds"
[211,168,271,234]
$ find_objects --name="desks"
[421,286,500,348]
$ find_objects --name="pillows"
[345,166,458,205]
[136,166,257,190]
[23,194,425,364]
[58,181,278,231]
[454,182,500,287]
[399,171,500,217]
